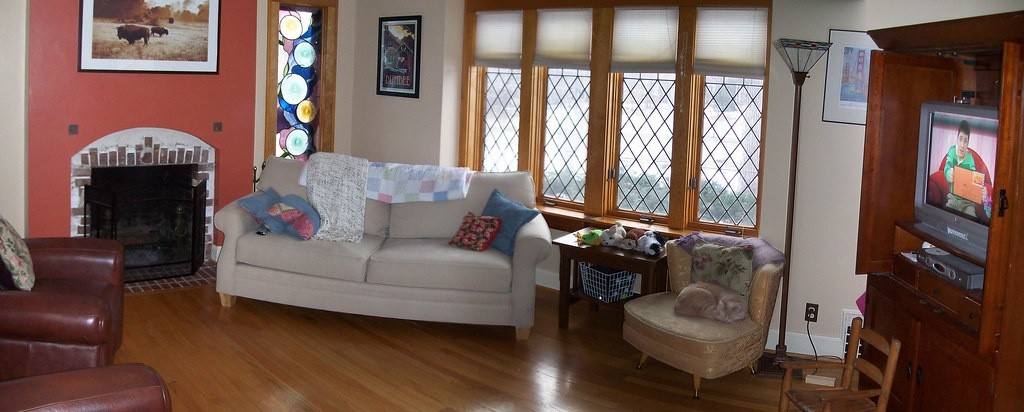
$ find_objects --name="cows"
[116,23,168,46]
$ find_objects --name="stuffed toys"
[581,222,664,258]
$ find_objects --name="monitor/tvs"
[913,100,999,262]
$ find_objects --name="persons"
[943,120,977,217]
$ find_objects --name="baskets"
[578,261,636,303]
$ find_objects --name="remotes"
[257,226,267,235]
